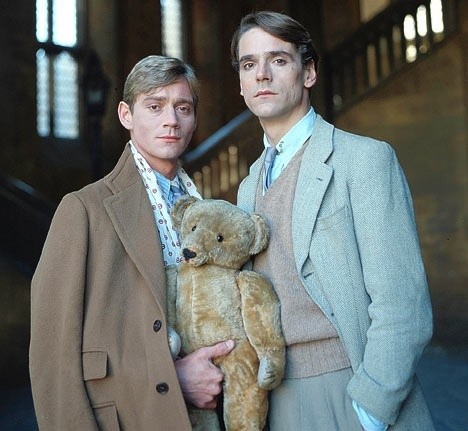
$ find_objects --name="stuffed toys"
[165,195,285,431]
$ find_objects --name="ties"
[263,147,278,196]
[170,181,186,203]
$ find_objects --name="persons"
[29,54,235,431]
[232,10,434,431]
